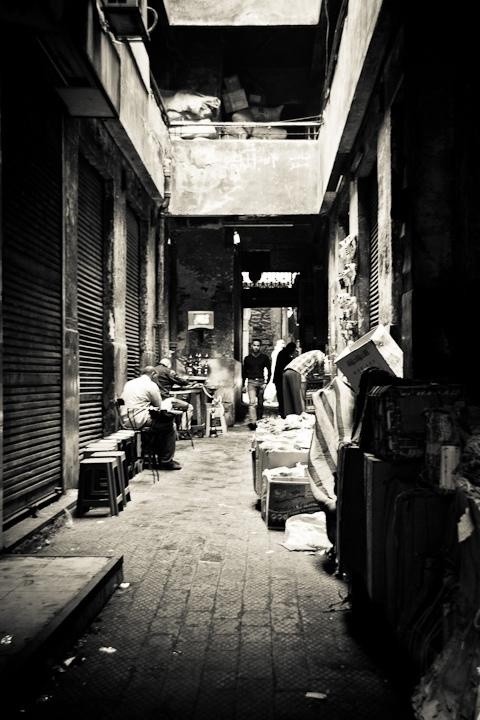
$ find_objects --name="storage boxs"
[260,469,320,531]
[333,324,404,395]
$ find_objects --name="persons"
[152,355,193,431]
[281,349,327,419]
[240,337,271,431]
[117,362,182,471]
[271,340,300,418]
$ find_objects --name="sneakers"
[159,460,182,471]
[179,431,192,440]
[248,422,257,431]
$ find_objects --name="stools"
[76,428,137,518]
